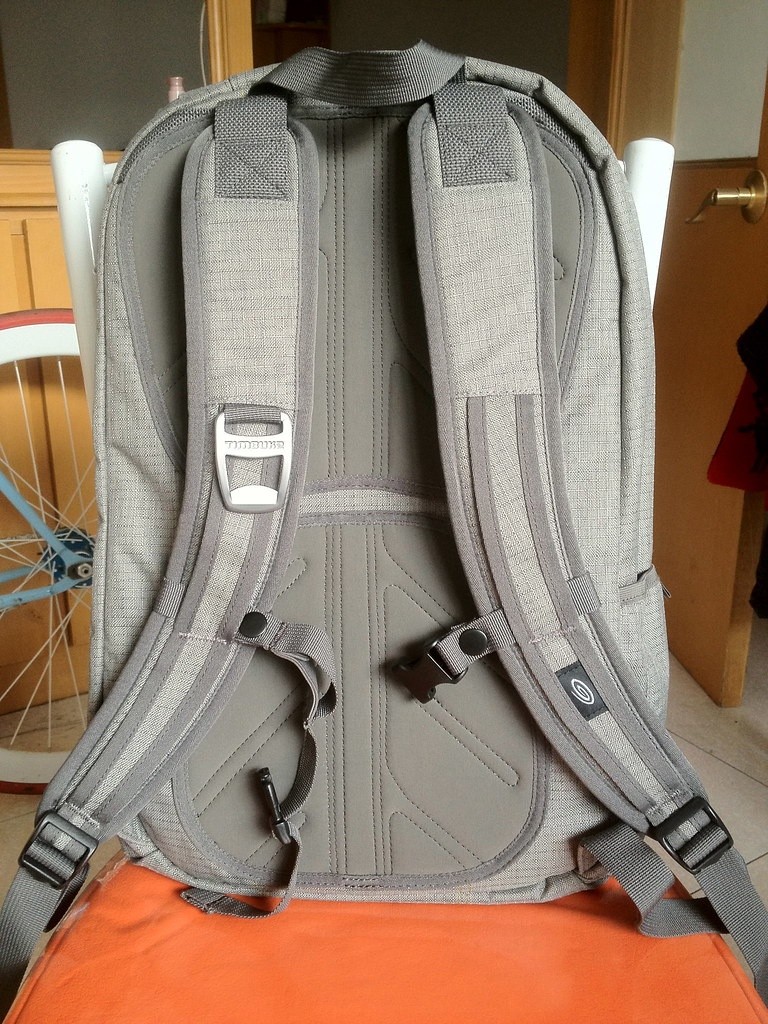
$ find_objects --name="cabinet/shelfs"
[1,148,126,715]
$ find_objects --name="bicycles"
[0,306,112,798]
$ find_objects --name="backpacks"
[0,42,767,1007]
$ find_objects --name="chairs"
[1,137,767,1024]
